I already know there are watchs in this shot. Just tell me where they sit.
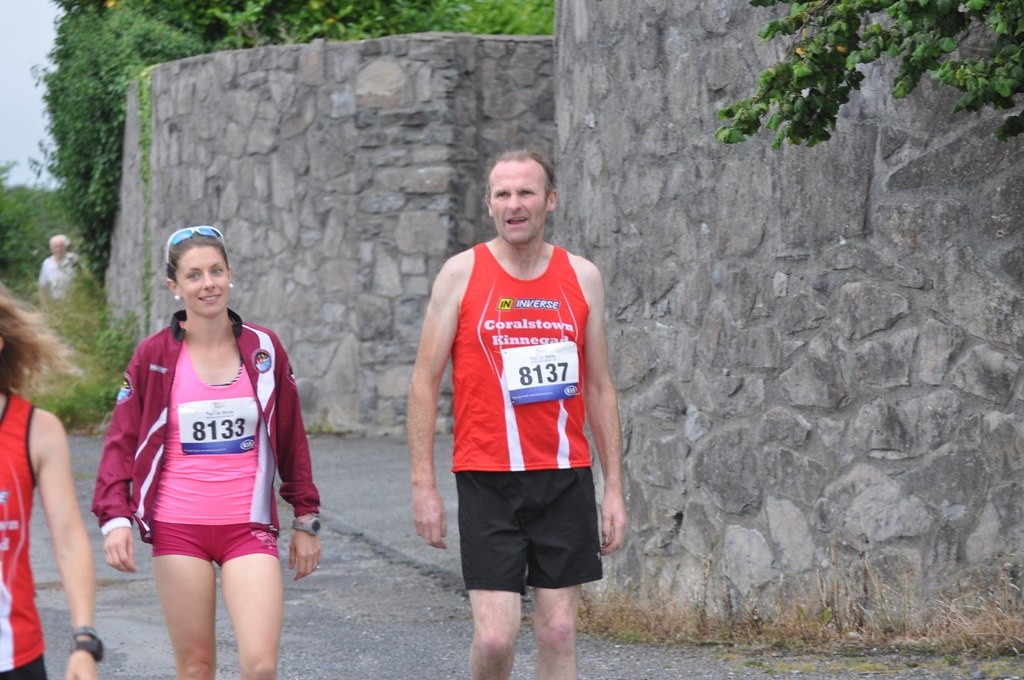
[68,625,103,661]
[290,516,322,535]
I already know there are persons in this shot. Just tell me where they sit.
[404,149,630,680]
[0,283,105,680]
[38,235,99,305]
[91,223,323,679]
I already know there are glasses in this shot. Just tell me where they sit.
[165,226,224,264]
